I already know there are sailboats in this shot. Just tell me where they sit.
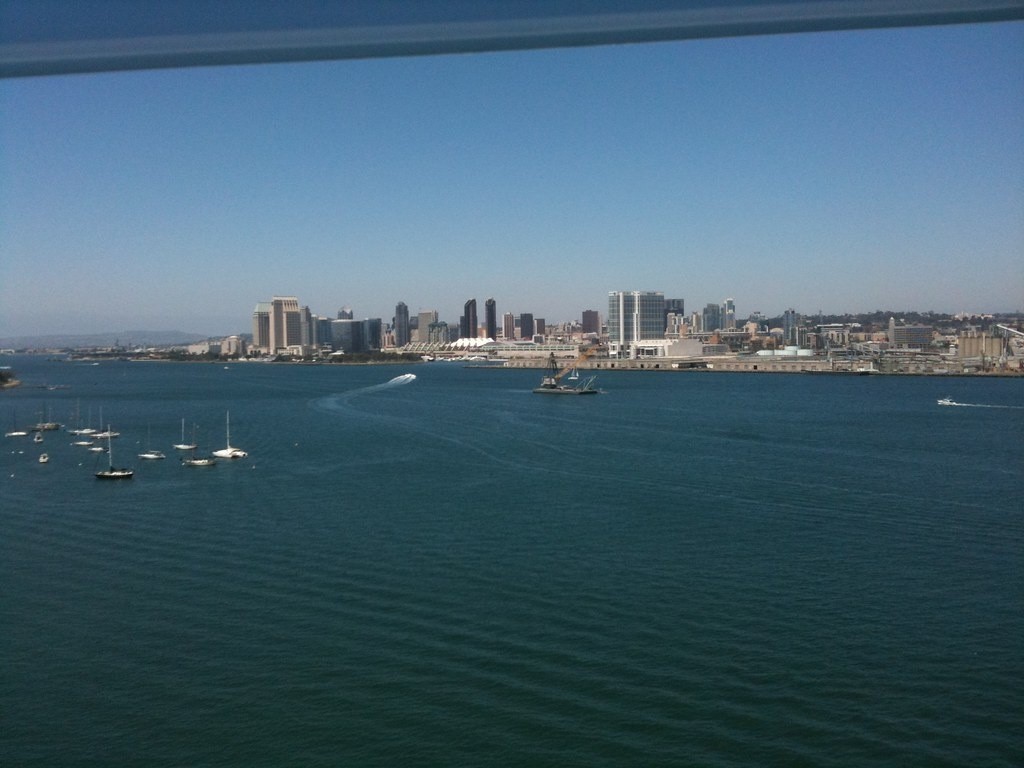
[5,400,251,479]
[567,365,580,380]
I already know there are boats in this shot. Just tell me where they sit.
[533,345,600,393]
